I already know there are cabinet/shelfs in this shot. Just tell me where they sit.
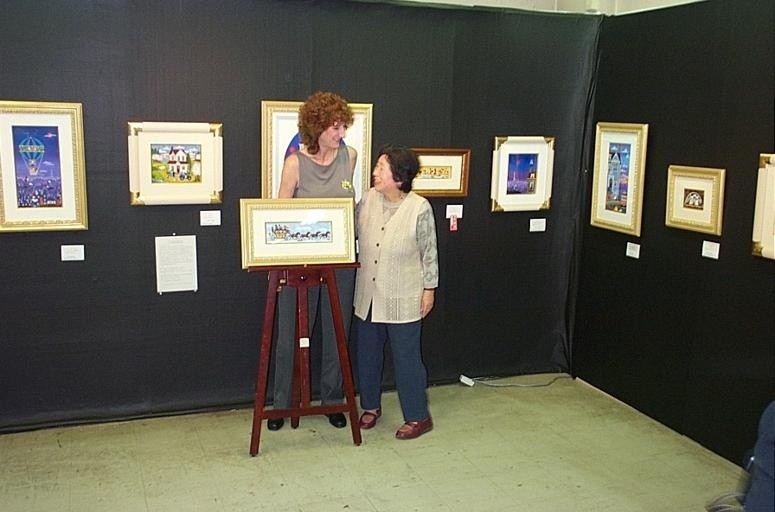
[246,261,362,458]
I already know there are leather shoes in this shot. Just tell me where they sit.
[267,418,284,430]
[360,405,381,429]
[325,413,346,427]
[396,418,433,439]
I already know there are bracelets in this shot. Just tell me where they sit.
[423,288,435,291]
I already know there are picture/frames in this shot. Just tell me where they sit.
[665,164,726,238]
[590,121,650,237]
[261,99,373,203]
[490,135,556,211]
[749,153,775,260]
[409,147,472,198]
[128,121,224,206]
[239,198,356,270]
[0,101,89,232]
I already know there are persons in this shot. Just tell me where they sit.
[352,143,439,440]
[266,89,358,431]
[741,401,775,512]
